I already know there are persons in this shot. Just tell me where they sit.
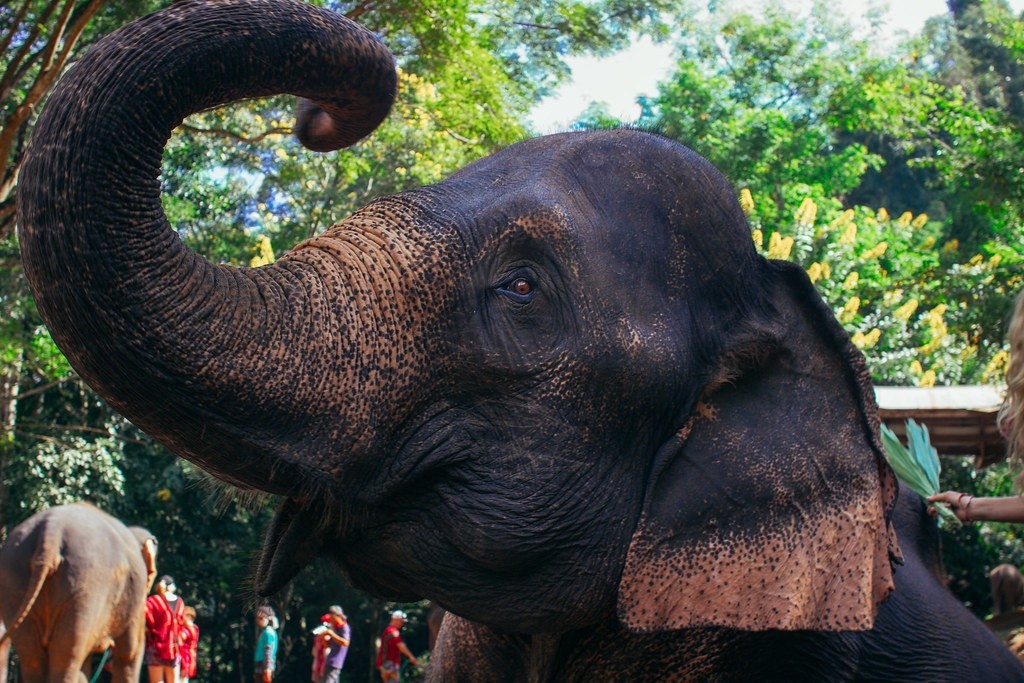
[923,285,1024,529]
[312,605,351,682]
[375,610,424,683]
[253,606,279,683]
[174,606,199,683]
[989,561,1024,666]
[310,614,335,683]
[144,575,185,683]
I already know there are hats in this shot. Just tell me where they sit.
[258,606,275,616]
[391,610,407,619]
[330,605,347,620]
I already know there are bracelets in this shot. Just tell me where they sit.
[958,493,974,523]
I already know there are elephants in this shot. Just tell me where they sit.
[0,500,159,683]
[17,1,1024,682]
